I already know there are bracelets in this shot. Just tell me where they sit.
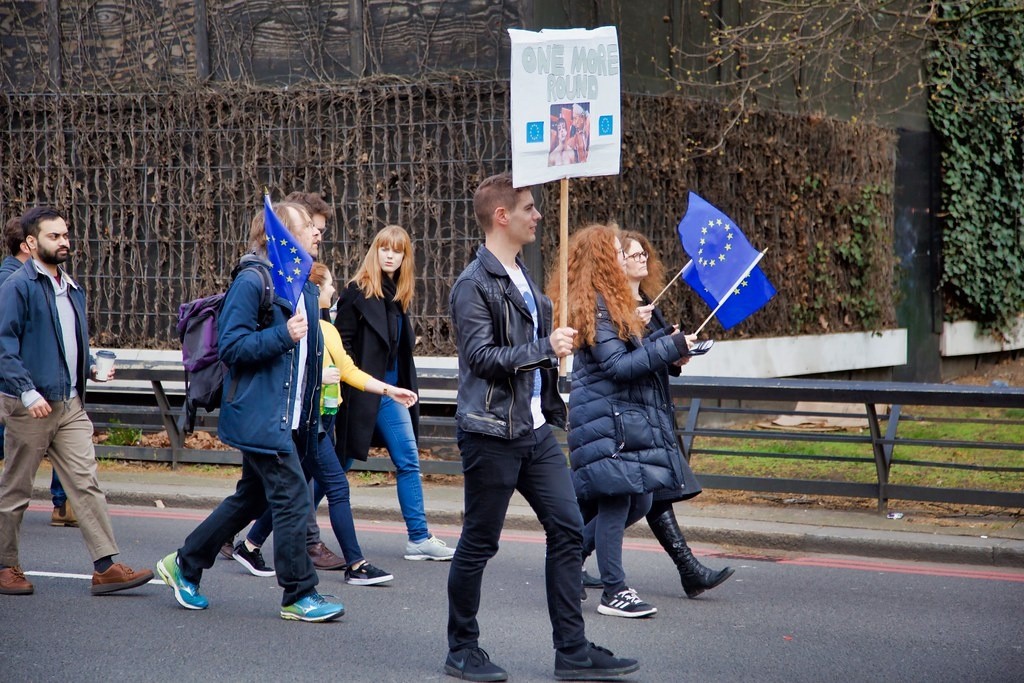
[383,385,388,395]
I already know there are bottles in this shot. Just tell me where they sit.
[323,365,338,415]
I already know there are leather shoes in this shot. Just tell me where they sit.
[307,541,346,568]
[0,565,33,592]
[91,562,154,592]
[220,538,234,559]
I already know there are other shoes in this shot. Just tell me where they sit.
[51,499,78,526]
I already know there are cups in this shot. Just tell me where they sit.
[96,350,116,381]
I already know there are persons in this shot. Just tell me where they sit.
[544,225,698,617]
[220,192,345,571]
[0,207,154,596]
[444,173,639,683]
[156,202,345,624]
[580,234,734,598]
[334,224,457,561]
[230,263,418,586]
[548,104,590,166]
[0,217,80,527]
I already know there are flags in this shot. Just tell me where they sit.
[678,190,759,303]
[263,195,314,317]
[682,262,775,329]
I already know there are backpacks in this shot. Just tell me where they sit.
[176,264,274,408]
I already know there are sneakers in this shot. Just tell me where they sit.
[444,647,508,681]
[281,592,345,622]
[598,586,657,618]
[156,549,208,609]
[580,587,588,603]
[345,561,394,584]
[403,534,457,561]
[554,641,640,679]
[232,542,276,576]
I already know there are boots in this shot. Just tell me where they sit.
[646,498,735,599]
[581,568,602,588]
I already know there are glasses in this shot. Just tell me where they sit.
[626,250,648,261]
[617,248,624,258]
